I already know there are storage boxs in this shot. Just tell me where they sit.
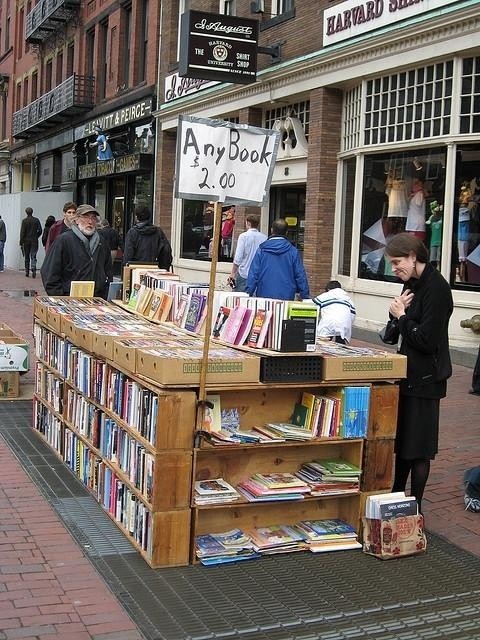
[0,324,30,398]
[31,294,407,382]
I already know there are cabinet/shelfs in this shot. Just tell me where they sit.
[195,384,372,568]
[358,385,403,541]
[32,321,192,572]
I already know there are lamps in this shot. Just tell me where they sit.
[412,153,423,171]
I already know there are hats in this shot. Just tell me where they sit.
[77,204,99,217]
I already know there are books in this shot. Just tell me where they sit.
[195,518,362,566]
[198,387,371,446]
[365,492,418,520]
[36,270,317,359]
[315,335,388,359]
[194,458,363,505]
[33,323,158,561]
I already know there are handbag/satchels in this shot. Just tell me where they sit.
[157,226,172,270]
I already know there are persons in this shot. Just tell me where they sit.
[20,207,42,278]
[230,214,268,294]
[46,201,76,253]
[310,281,357,346]
[384,166,475,264]
[89,127,114,162]
[379,233,454,518]
[245,219,311,302]
[462,464,480,514]
[96,219,119,251]
[122,206,173,271]
[0,220,7,272]
[466,243,479,396]
[40,203,114,299]
[41,216,56,247]
